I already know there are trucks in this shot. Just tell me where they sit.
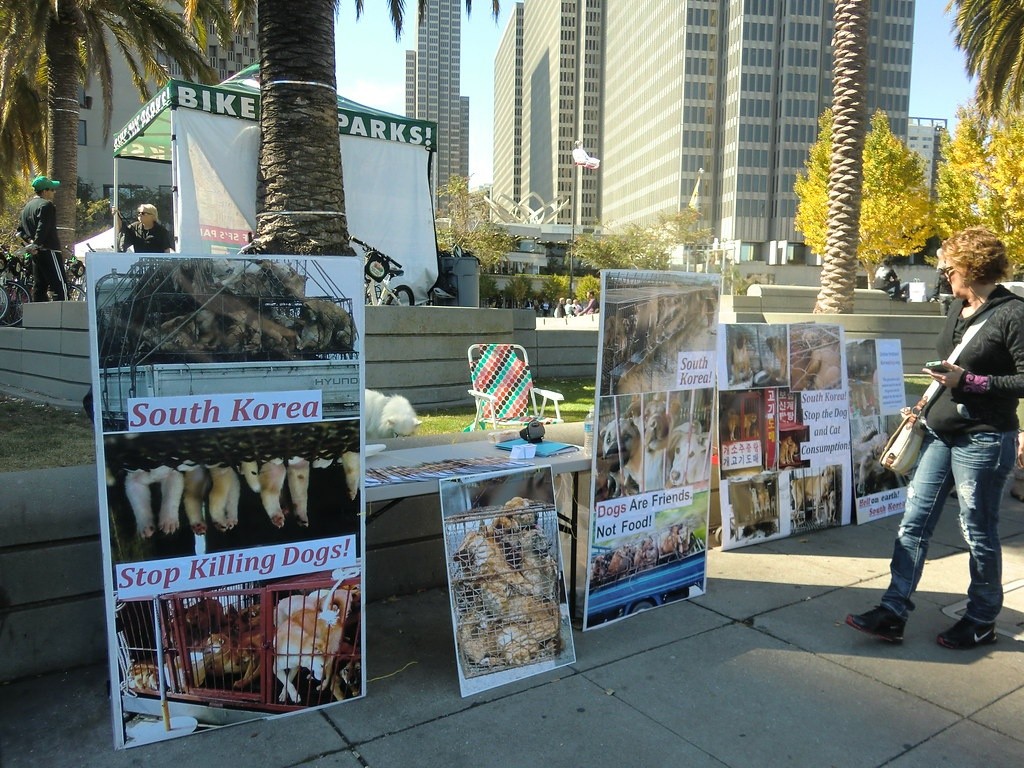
[98,357,360,421]
[587,548,704,617]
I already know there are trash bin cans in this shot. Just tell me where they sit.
[439,254,479,308]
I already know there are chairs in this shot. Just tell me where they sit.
[466,343,566,432]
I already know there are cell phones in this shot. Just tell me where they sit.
[926,360,952,373]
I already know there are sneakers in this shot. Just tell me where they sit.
[937,616,996,648]
[847,605,907,644]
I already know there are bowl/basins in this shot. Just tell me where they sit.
[753,370,773,387]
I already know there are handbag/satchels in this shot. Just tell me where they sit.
[880,409,926,476]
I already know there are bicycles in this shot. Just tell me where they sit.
[0,242,88,327]
[348,236,415,307]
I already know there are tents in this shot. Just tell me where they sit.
[114,65,441,305]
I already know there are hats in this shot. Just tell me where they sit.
[32,176,60,191]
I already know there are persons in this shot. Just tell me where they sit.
[112,204,175,255]
[528,292,599,316]
[874,238,951,302]
[846,227,1024,649]
[17,177,69,301]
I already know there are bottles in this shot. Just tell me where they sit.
[583,404,594,457]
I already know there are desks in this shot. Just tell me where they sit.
[363,440,592,621]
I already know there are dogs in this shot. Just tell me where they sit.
[586,275,718,631]
[729,436,840,544]
[125,588,361,708]
[452,497,561,666]
[728,408,756,441]
[365,388,423,438]
[725,324,843,391]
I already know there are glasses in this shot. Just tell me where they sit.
[137,211,154,216]
[944,265,955,280]
